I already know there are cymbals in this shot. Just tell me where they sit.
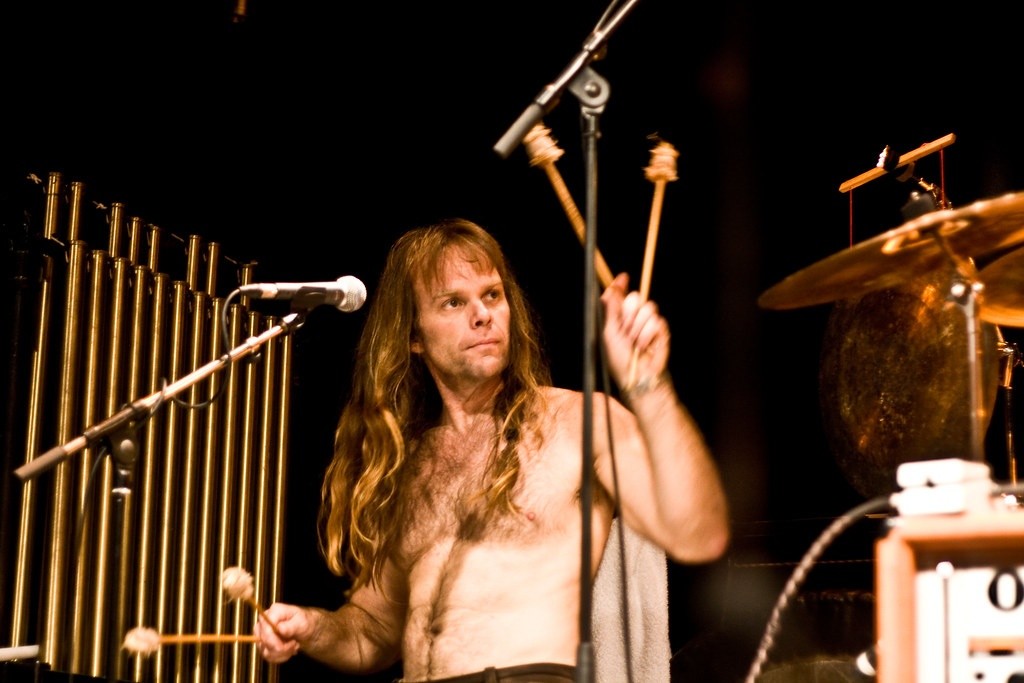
[978,244,1024,328]
[756,188,1024,309]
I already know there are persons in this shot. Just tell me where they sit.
[252,223,730,683]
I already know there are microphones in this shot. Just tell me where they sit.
[239,276,367,313]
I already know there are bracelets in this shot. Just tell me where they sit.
[620,371,670,405]
